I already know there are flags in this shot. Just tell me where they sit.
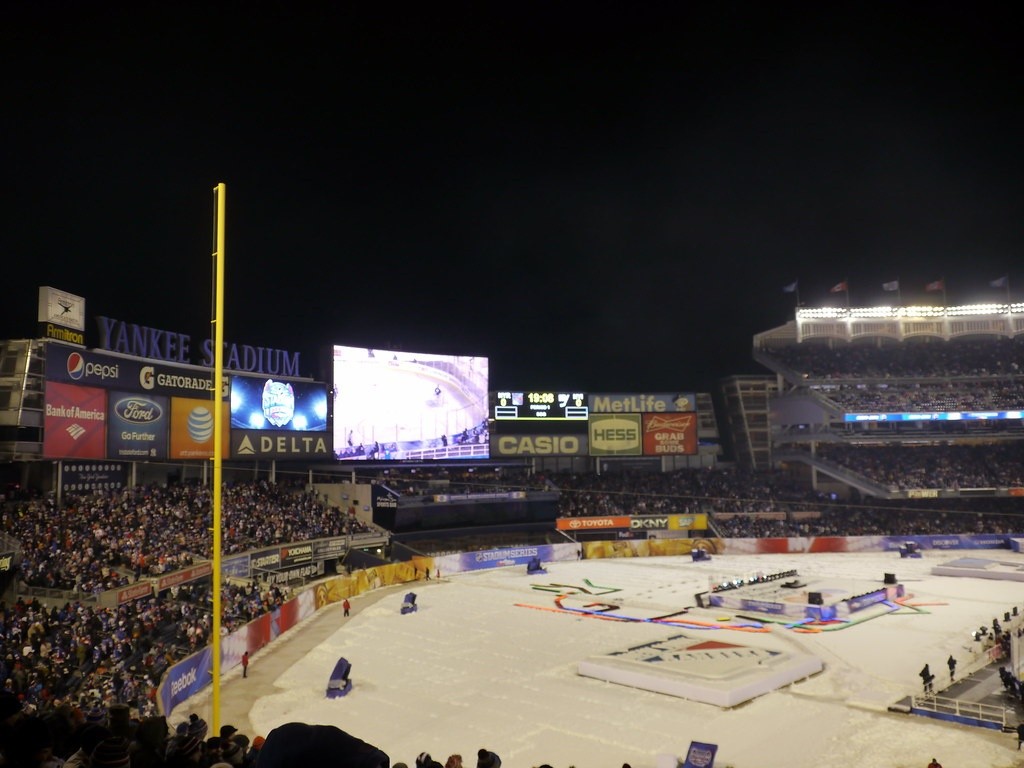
[784,282,797,292]
[926,280,942,291]
[832,281,846,292]
[883,281,898,291]
[991,276,1007,288]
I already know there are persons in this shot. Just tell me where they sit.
[333,355,489,460]
[759,337,1024,488]
[242,651,250,677]
[343,599,350,617]
[0,465,1024,768]
[919,663,933,694]
[947,654,957,681]
[577,549,581,562]
[426,567,431,581]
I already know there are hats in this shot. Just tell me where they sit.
[253,736,266,750]
[221,725,238,739]
[175,734,201,756]
[86,707,105,723]
[89,737,132,768]
[221,740,242,761]
[477,749,501,768]
[188,714,207,736]
[0,690,24,719]
[11,717,54,753]
